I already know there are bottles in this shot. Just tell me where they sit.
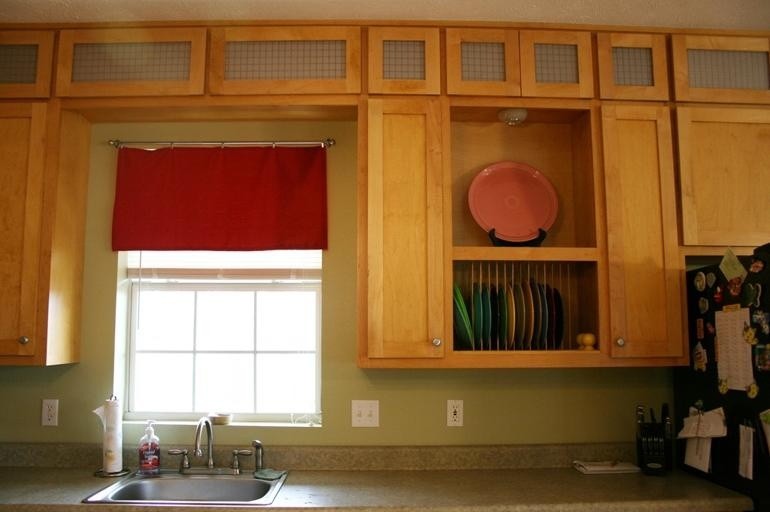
[138,421,162,475]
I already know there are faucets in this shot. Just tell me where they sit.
[194,417,214,469]
[251,440,264,474]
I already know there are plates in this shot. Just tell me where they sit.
[468,161,558,242]
[453,279,574,349]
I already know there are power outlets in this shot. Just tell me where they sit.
[40,398,61,427]
[444,399,465,428]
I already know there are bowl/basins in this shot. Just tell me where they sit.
[208,412,232,425]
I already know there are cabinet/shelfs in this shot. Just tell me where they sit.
[55,20,366,125]
[670,28,770,258]
[0,24,92,368]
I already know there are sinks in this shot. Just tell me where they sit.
[81,468,289,506]
